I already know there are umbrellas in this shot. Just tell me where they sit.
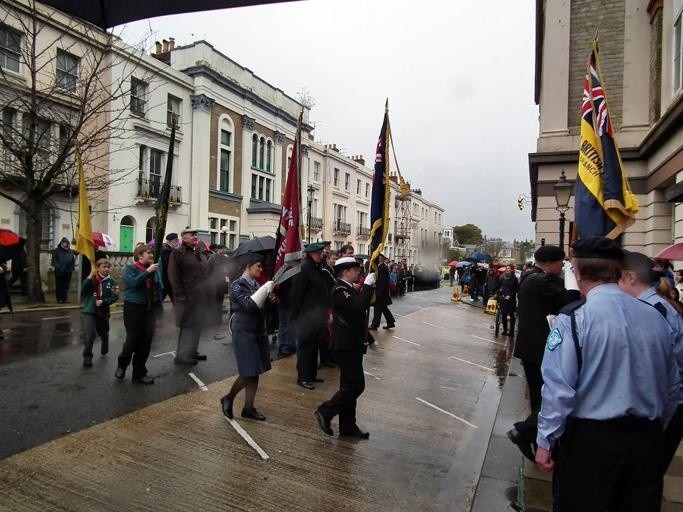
[653,242,683,261]
[0,229,21,246]
[447,251,492,269]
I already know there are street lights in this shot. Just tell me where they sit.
[552,172,572,251]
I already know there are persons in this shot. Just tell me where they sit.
[535,237,678,512]
[506,244,586,463]
[449,263,544,336]
[83,228,231,383]
[384,256,441,298]
[0,237,75,320]
[617,252,683,476]
[651,258,683,318]
[219,240,396,439]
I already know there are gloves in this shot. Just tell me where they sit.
[250,280,275,309]
[504,295,511,302]
[363,273,376,286]
[177,294,186,306]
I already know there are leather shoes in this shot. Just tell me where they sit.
[506,428,535,463]
[191,352,206,360]
[58,300,63,304]
[383,323,395,329]
[132,376,153,384]
[314,410,333,435]
[217,305,227,313]
[297,379,314,389]
[349,429,369,438]
[174,356,197,366]
[241,408,265,421]
[368,326,377,330]
[323,362,336,368]
[279,344,291,356]
[311,376,323,382]
[221,396,234,419]
[63,300,71,303]
[115,368,125,379]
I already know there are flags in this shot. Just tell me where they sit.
[271,114,307,287]
[367,112,390,273]
[153,126,176,263]
[574,35,639,240]
[75,158,97,273]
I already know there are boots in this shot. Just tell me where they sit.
[501,316,507,336]
[507,318,515,336]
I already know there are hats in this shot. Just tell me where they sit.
[304,243,324,252]
[534,246,565,262]
[379,254,385,258]
[332,257,360,271]
[166,233,177,240]
[570,237,624,262]
[209,244,218,250]
[218,243,224,249]
[181,229,198,237]
[622,250,656,270]
[239,253,265,264]
[322,241,331,246]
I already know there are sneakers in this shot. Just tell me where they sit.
[101,342,108,354]
[84,357,92,366]
[369,341,378,350]
